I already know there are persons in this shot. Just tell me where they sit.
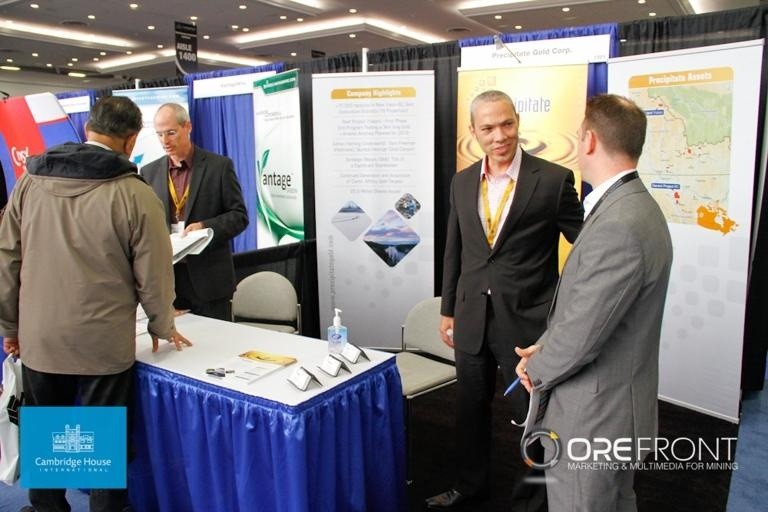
[423,88,585,510]
[139,101,251,322]
[0,94,193,511]
[514,94,675,510]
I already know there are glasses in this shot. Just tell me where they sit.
[155,128,178,138]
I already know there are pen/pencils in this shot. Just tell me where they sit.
[504,369,528,396]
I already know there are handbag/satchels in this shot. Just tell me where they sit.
[0,351,28,485]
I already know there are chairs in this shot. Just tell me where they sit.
[230,271,302,337]
[394,296,461,461]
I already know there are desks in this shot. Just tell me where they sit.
[75,305,409,512]
